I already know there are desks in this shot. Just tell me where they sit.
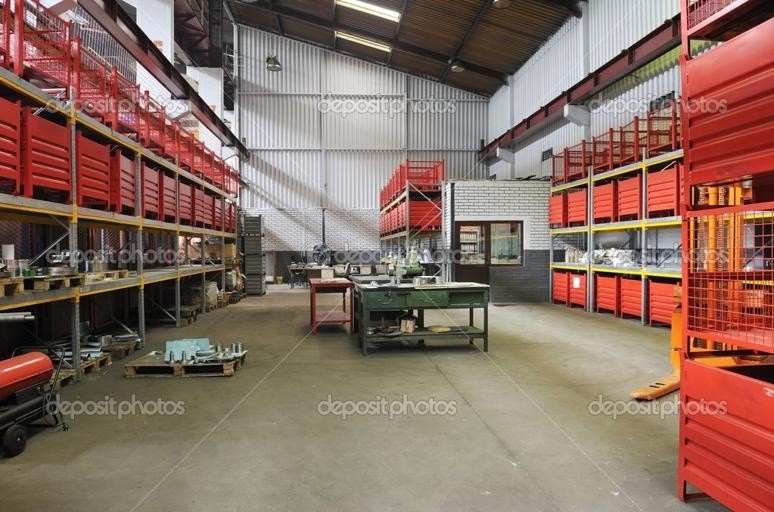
[355,282,490,356]
[549,147,774,326]
[311,278,355,335]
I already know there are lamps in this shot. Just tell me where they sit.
[266,10,281,71]
[451,53,464,73]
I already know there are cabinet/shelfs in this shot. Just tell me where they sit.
[0,66,238,385]
[379,180,446,282]
